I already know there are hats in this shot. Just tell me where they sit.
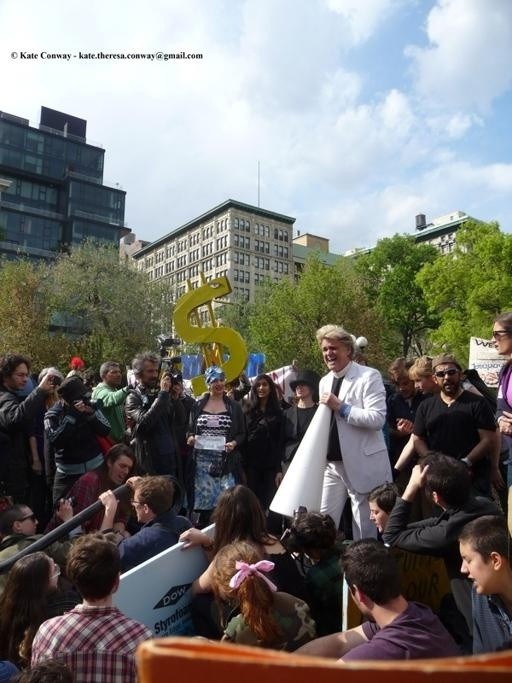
[289,369,320,393]
[204,366,225,385]
[431,352,462,371]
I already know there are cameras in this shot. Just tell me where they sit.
[82,397,103,410]
[50,376,58,385]
[165,373,182,387]
[286,506,307,552]
[56,496,78,512]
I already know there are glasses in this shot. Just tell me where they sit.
[435,369,458,378]
[50,562,62,580]
[492,330,506,337]
[21,512,36,524]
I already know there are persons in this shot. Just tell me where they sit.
[3,312,512,683]
